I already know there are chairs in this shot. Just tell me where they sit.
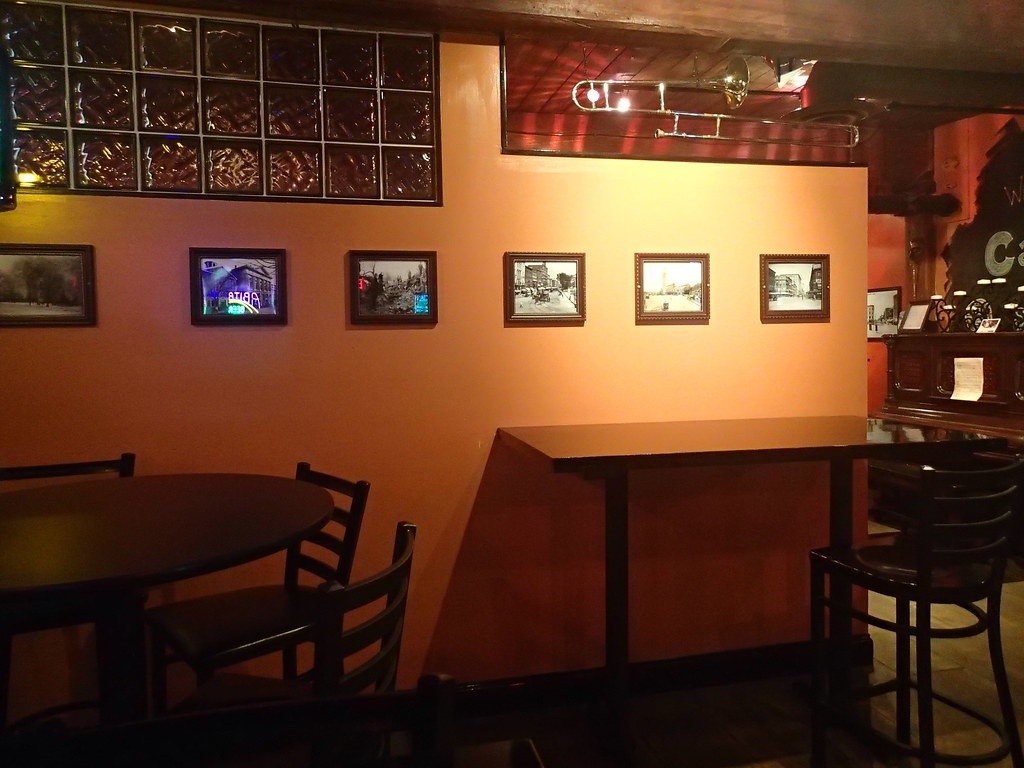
[807,454,1024,768]
[145,461,370,717]
[124,521,417,768]
[1,451,134,710]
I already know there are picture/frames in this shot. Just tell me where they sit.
[635,251,711,326]
[760,253,830,323]
[189,246,288,327]
[348,250,438,325]
[867,286,903,341]
[504,251,586,325]
[0,242,97,327]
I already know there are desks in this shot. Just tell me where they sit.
[0,472,335,768]
[499,418,1009,767]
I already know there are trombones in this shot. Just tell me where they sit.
[572,57,859,148]
